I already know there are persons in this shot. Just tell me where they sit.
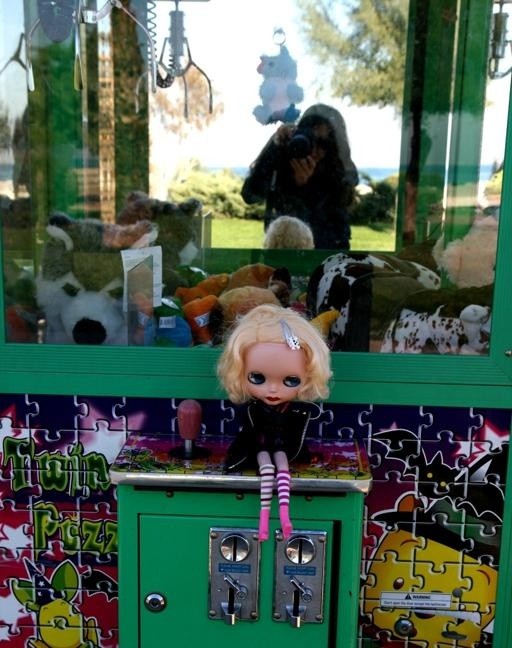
[217,304,335,543]
[241,99,357,249]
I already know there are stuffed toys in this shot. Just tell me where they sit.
[5,191,499,355]
[252,44,303,125]
[6,192,499,356]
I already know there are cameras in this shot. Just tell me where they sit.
[282,126,314,163]
